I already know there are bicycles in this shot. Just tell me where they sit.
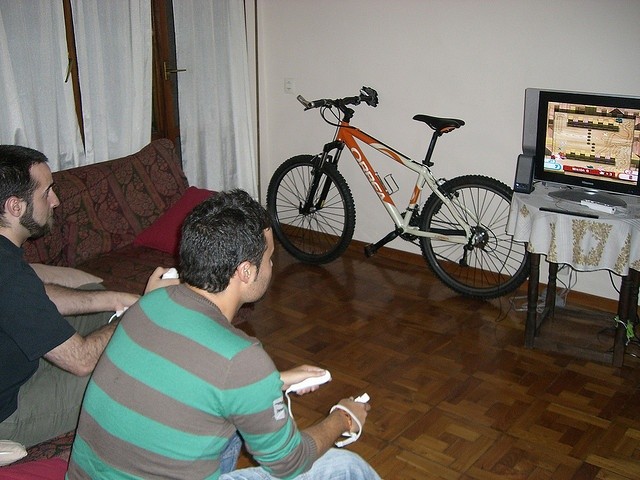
[267,86,532,298]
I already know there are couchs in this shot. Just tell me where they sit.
[0,428,76,480]
[23,139,225,296]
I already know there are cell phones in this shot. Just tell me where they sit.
[513,154,535,194]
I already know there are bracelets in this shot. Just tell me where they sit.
[337,406,354,435]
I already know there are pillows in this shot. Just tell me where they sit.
[0,458,68,480]
[135,187,219,252]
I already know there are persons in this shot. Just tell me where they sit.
[62,190,385,479]
[0,142,182,458]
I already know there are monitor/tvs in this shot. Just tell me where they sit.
[533,91,639,207]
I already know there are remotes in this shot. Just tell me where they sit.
[580,199,617,214]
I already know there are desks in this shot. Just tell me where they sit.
[507,182,640,371]
